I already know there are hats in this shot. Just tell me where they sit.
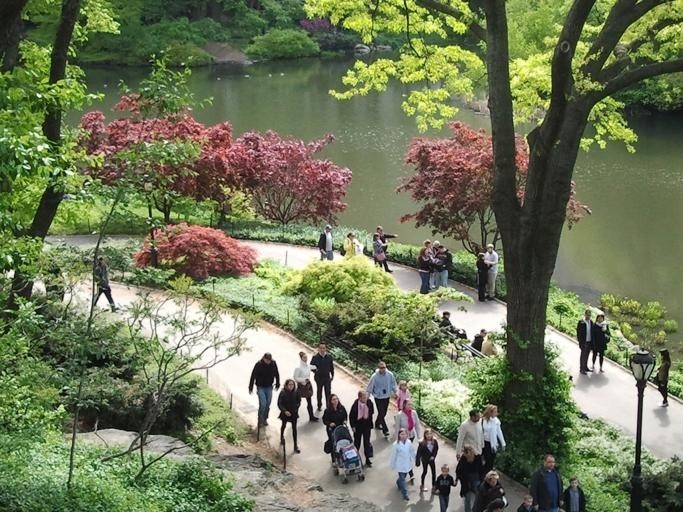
[484,242,495,250]
[323,225,333,230]
[432,239,440,248]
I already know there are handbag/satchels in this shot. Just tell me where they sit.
[324,438,332,453]
[599,332,611,343]
[297,381,313,398]
[375,251,388,261]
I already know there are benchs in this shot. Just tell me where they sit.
[439,329,466,361]
[457,342,486,364]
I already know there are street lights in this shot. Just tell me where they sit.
[140,173,159,271]
[626,346,655,512]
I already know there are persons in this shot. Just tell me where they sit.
[91,257,119,312]
[373,233,393,272]
[476,253,492,302]
[656,349,671,407]
[376,226,398,267]
[417,240,452,294]
[343,232,361,261]
[318,225,333,260]
[484,243,498,301]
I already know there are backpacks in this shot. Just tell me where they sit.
[339,239,349,255]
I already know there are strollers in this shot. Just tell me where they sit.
[323,422,365,485]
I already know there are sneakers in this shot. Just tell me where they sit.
[111,307,120,312]
[395,476,439,501]
[330,459,374,469]
[475,294,495,304]
[384,268,394,274]
[280,438,302,453]
[419,284,437,294]
[308,407,322,422]
[255,418,270,428]
[577,365,608,377]
[374,426,390,437]
[658,402,669,408]
